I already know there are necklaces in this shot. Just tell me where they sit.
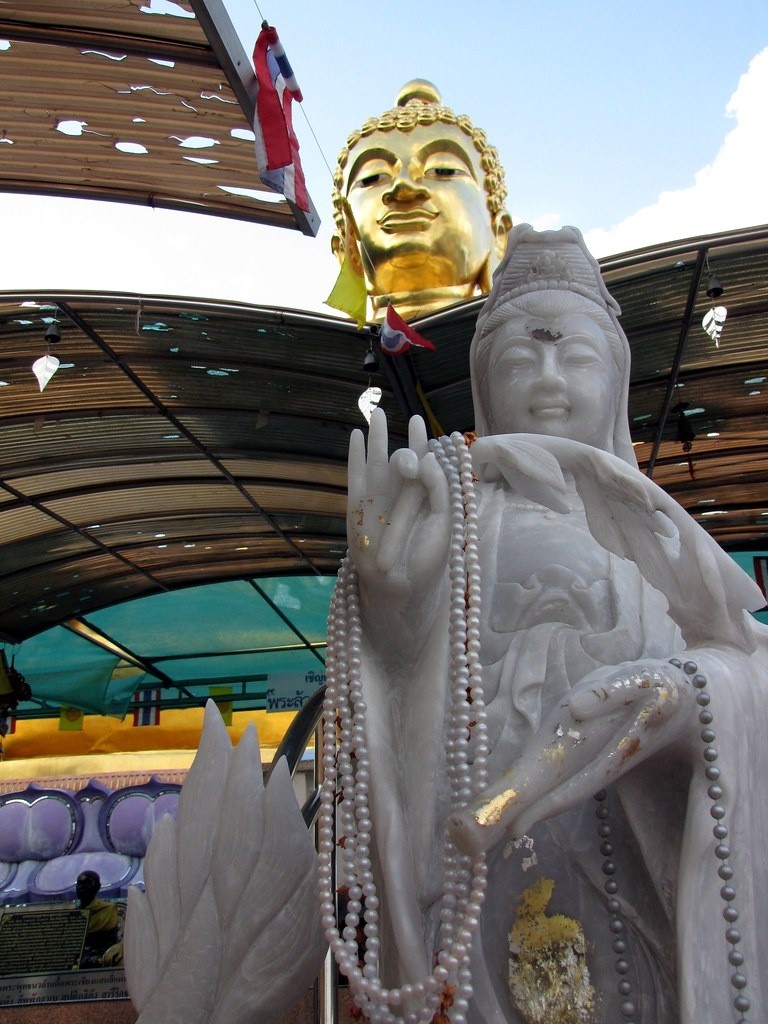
[317,429,490,1024]
[595,657,751,1024]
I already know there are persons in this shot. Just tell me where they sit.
[331,77,512,322]
[342,224,767,1024]
[73,870,123,968]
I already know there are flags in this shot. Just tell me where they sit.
[253,22,312,215]
[380,301,436,355]
[324,209,367,329]
